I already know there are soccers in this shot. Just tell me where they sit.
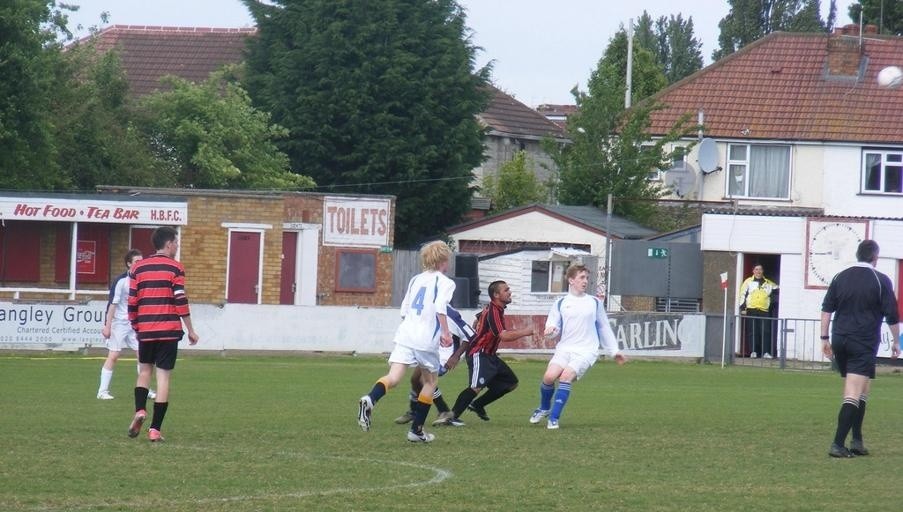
[878,65,902,89]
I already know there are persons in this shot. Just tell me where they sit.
[740,263,779,359]
[97,248,156,399]
[358,240,456,442]
[821,240,901,458]
[394,302,478,424]
[529,265,626,430]
[450,281,535,426]
[127,227,199,442]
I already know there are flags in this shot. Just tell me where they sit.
[720,272,728,289]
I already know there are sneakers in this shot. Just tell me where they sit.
[829,442,853,457]
[148,390,157,401]
[128,410,148,438]
[547,415,560,431]
[395,401,490,443]
[97,392,115,400]
[529,407,551,424]
[148,428,161,442]
[763,352,772,358]
[750,352,757,359]
[850,440,869,456]
[358,395,373,432]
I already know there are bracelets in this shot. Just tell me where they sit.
[820,335,829,340]
